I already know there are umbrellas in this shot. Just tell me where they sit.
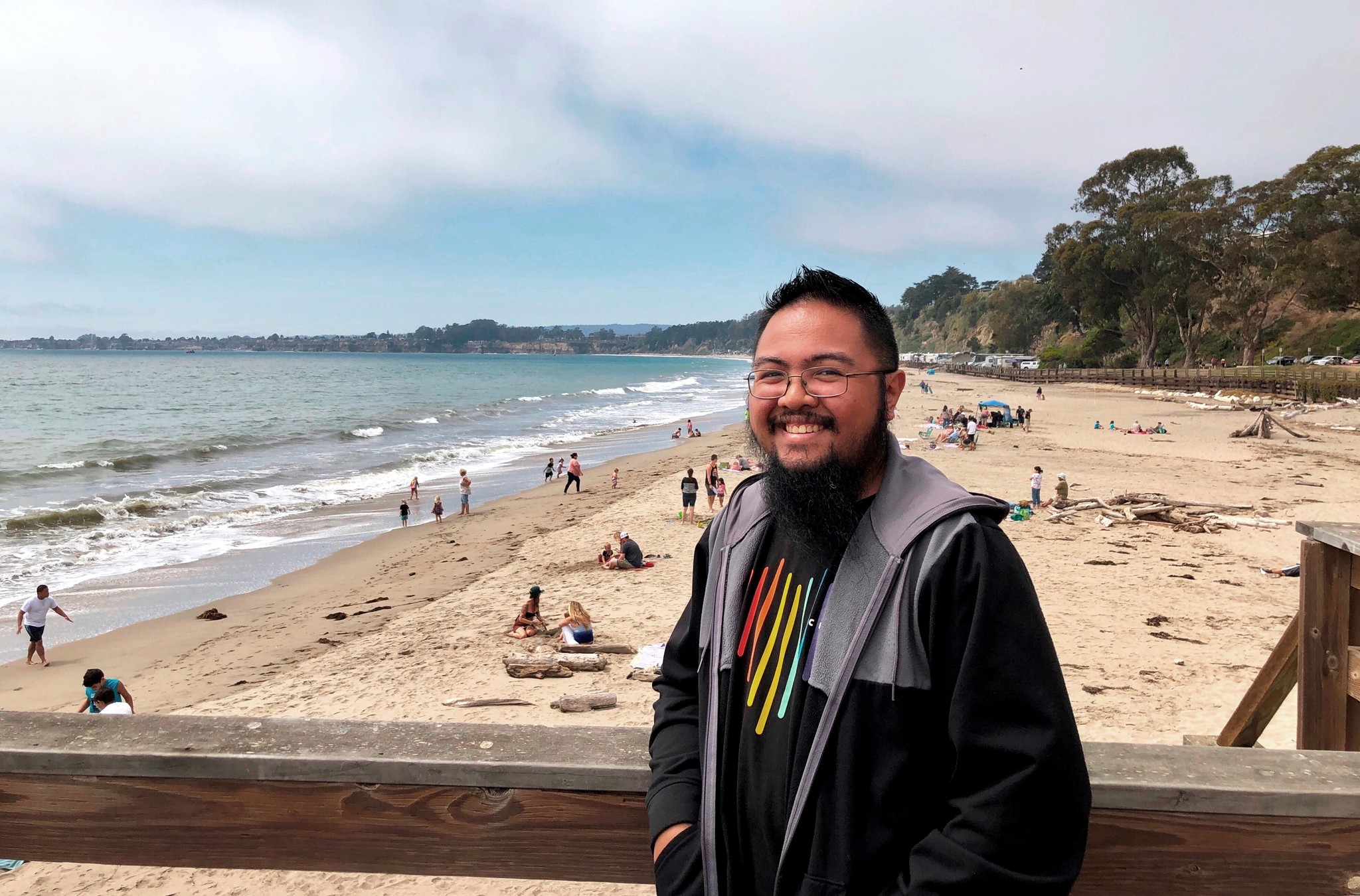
[1007,358,1017,369]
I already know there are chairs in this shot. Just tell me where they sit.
[947,438,959,443]
[918,427,933,439]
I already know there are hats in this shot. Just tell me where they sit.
[948,423,953,427]
[620,532,629,539]
[560,457,564,459]
[961,412,965,415]
[529,586,543,595]
[1057,472,1066,480]
[735,455,739,459]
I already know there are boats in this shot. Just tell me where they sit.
[186,350,195,353]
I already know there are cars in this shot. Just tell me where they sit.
[1312,356,1350,366]
[1345,355,1360,365]
[1266,356,1297,366]
[1296,356,1324,365]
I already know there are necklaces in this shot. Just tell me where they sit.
[38,596,45,606]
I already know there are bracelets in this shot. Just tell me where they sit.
[542,620,545,621]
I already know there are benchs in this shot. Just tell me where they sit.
[1203,363,1212,369]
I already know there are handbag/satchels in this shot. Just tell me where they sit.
[1010,514,1023,520]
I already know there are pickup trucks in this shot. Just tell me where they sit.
[1020,361,1040,370]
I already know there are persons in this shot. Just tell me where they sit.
[564,453,583,495]
[431,496,444,524]
[556,601,594,645]
[892,380,1164,450]
[644,262,1094,896]
[457,469,471,516]
[611,468,620,489]
[409,476,420,500]
[94,686,132,714]
[556,457,566,479]
[671,407,756,525]
[598,531,643,570]
[1059,362,1067,370]
[1145,355,1225,370]
[1029,466,1044,510]
[77,669,135,714]
[399,499,410,527]
[16,585,73,666]
[1041,472,1068,507]
[543,458,556,482]
[503,586,546,640]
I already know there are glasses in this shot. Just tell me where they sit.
[743,366,894,399]
